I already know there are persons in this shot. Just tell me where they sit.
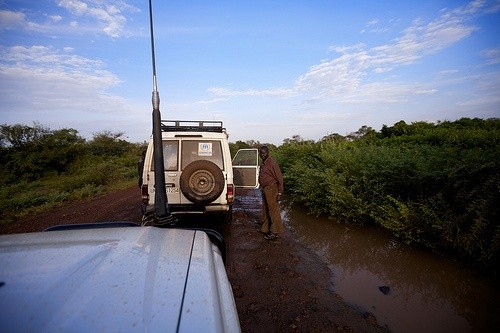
[257,146,283,239]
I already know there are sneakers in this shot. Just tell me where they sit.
[256,228,262,232]
[263,233,279,239]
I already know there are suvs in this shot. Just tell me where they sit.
[139,120,258,217]
